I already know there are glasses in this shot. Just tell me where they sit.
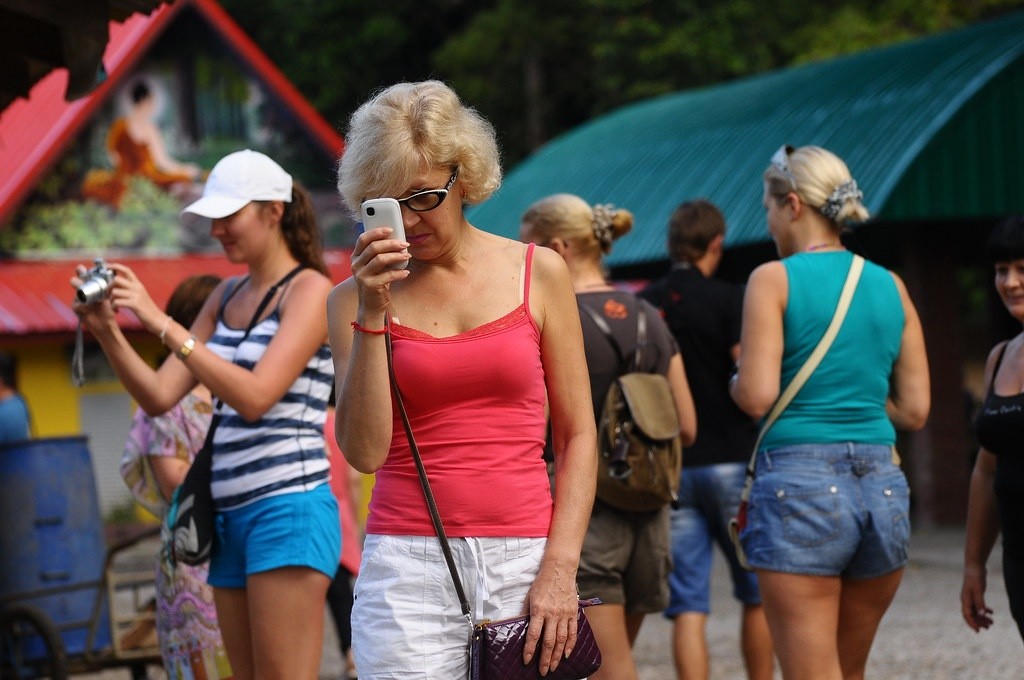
[362,162,461,211]
[770,144,796,198]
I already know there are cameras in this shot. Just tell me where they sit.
[77,258,117,306]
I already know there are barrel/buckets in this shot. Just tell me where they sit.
[0,433,113,661]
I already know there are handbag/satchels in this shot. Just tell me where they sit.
[170,444,215,565]
[727,470,757,573]
[469,599,605,680]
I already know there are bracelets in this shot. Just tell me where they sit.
[177,337,200,361]
[159,316,174,345]
[352,321,388,334]
[728,374,738,384]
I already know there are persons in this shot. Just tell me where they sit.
[730,143,932,680]
[119,274,235,680]
[71,149,342,680]
[634,198,762,680]
[960,206,1024,641]
[326,79,599,680]
[521,194,697,680]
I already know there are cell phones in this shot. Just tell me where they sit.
[361,198,409,273]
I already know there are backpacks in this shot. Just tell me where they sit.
[574,294,680,515]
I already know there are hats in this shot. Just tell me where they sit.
[179,145,292,222]
